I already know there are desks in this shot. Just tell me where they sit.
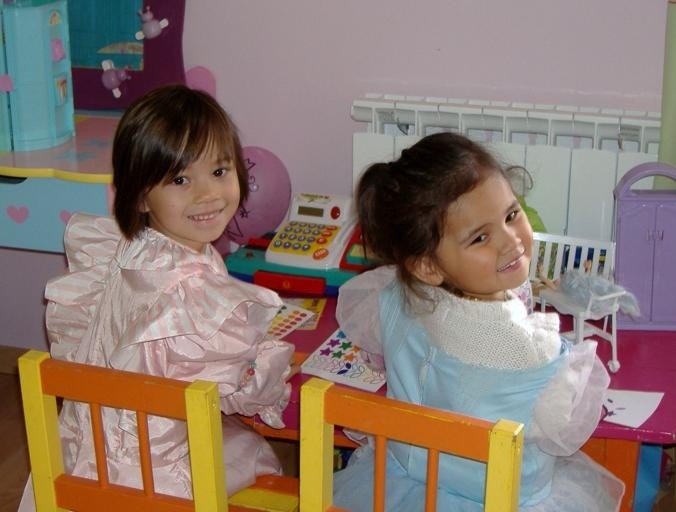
[199,264,673,510]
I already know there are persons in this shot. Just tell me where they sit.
[16,86,295,511]
[334,132,626,511]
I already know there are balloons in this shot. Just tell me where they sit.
[217,145,292,246]
